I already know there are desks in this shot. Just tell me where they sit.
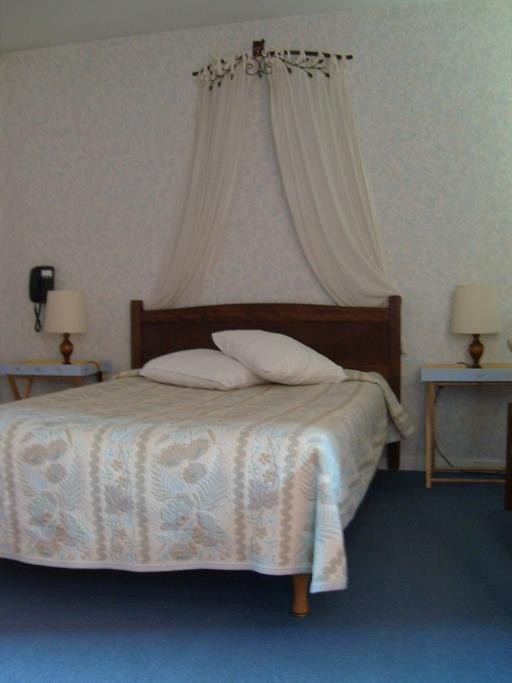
[1,359,111,400]
[418,362,512,489]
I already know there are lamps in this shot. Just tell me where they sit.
[44,289,91,364]
[449,282,502,368]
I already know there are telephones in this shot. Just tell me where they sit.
[29,266,54,303]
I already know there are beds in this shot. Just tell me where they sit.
[1,295,403,618]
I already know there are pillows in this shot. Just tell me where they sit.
[138,327,347,393]
